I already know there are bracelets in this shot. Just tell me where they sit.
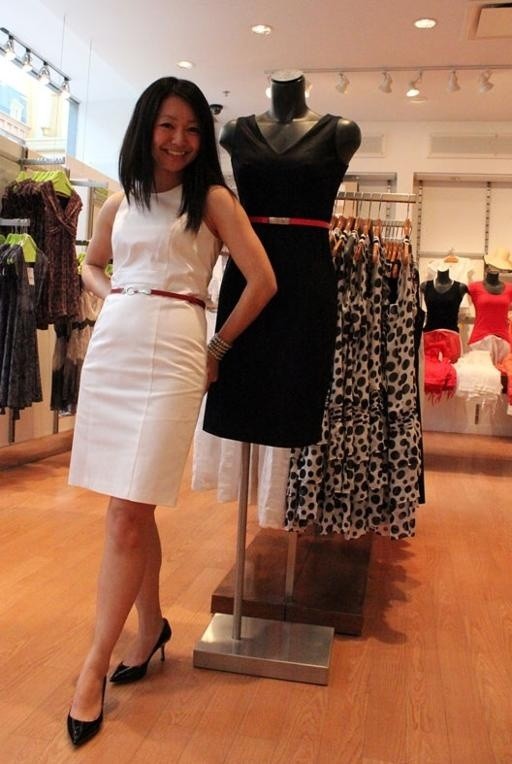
[208,333,231,361]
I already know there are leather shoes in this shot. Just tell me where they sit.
[66,675,107,746]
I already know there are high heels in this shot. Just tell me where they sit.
[108,617,173,684]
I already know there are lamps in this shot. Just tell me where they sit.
[262,64,501,102]
[0,25,72,96]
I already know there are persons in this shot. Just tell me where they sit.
[201,70,361,448]
[419,267,467,363]
[67,77,278,748]
[466,270,512,366]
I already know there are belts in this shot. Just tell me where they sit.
[110,287,207,311]
[247,216,331,230]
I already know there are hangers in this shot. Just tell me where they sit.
[319,188,420,280]
[71,239,116,278]
[0,217,52,266]
[430,246,472,266]
[8,157,82,204]
